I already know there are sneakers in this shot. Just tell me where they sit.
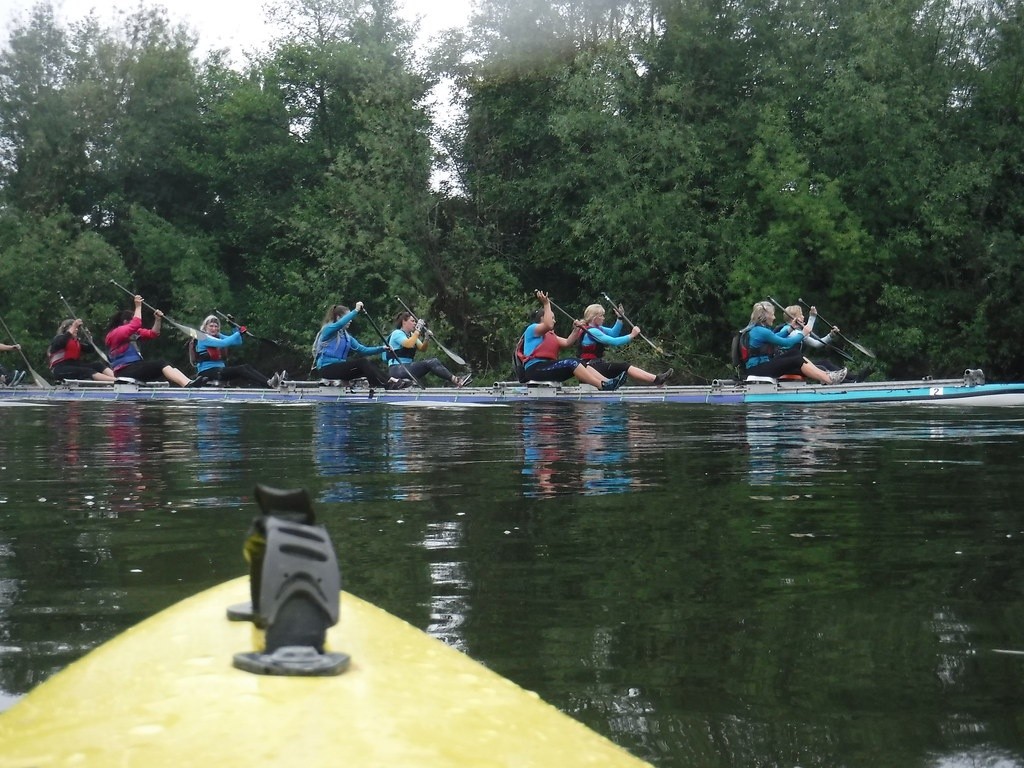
[4,370,26,386]
[600,371,626,391]
[828,367,847,385]
[185,376,209,388]
[268,370,288,388]
[386,378,412,389]
[654,368,673,387]
[458,372,474,387]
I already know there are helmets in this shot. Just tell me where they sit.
[61,319,74,326]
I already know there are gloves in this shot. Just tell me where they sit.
[424,330,433,340]
[226,314,236,328]
[415,319,426,331]
[240,326,247,333]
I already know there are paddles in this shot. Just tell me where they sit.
[109,278,208,342]
[0,316,52,389]
[57,290,112,366]
[211,306,280,348]
[393,294,467,365]
[360,306,427,392]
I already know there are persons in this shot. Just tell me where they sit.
[48,319,116,381]
[0,343,27,388]
[742,301,847,386]
[105,294,209,388]
[524,291,626,391]
[316,301,413,390]
[387,311,473,388]
[197,314,287,389]
[580,303,674,387]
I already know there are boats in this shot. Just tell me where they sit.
[2,374,1024,406]
